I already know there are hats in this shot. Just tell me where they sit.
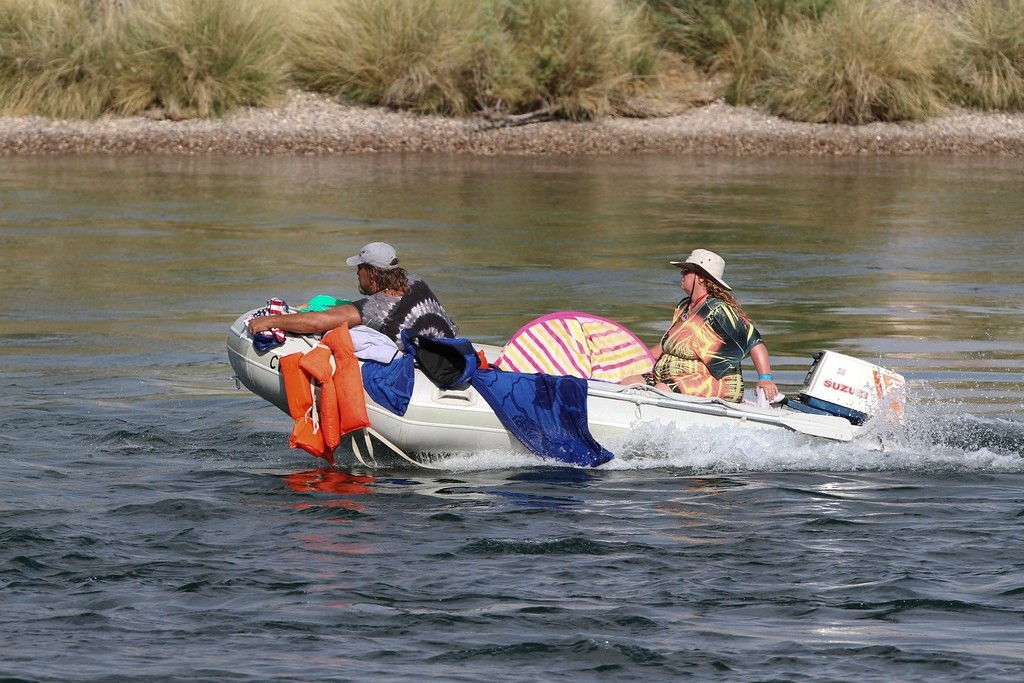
[345,241,400,270]
[669,249,733,291]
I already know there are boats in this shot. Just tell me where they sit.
[227,304,895,471]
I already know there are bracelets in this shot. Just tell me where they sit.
[758,374,774,380]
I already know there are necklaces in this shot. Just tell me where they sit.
[681,293,708,322]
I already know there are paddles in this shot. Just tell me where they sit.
[587,386,855,442]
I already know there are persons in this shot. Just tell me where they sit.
[619,249,779,402]
[249,242,459,354]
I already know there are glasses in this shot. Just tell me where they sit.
[679,266,693,276]
